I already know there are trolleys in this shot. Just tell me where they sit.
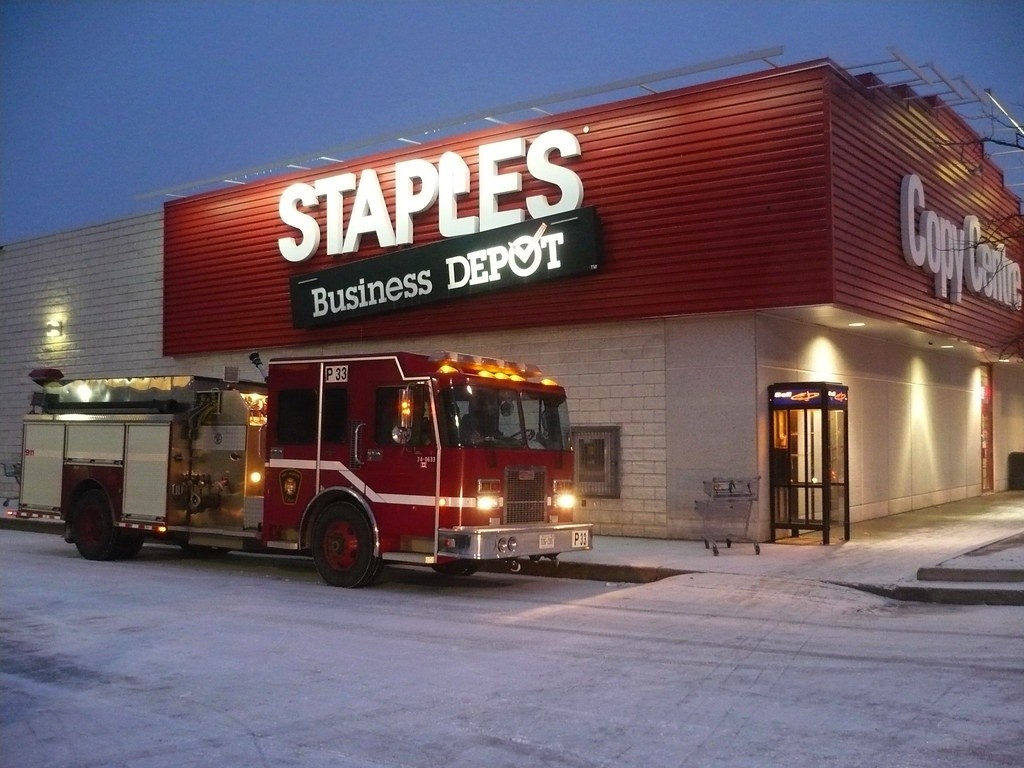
[693,473,762,556]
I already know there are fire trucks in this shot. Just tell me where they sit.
[16,349,597,590]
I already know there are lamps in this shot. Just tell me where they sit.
[46,321,62,337]
